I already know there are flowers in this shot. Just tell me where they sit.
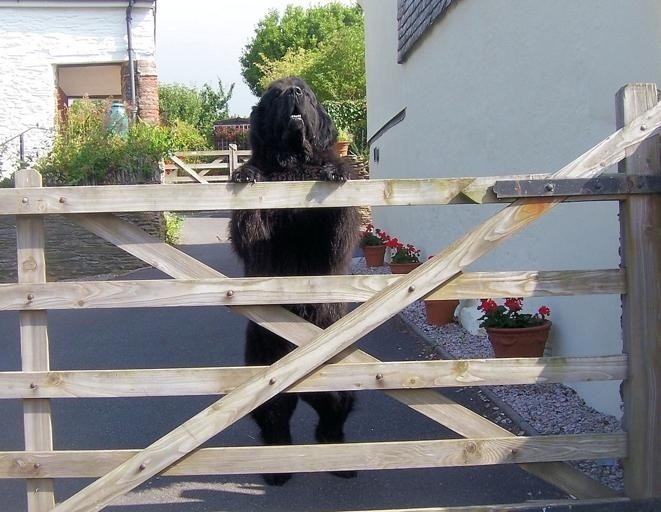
[478,297,552,329]
[358,224,421,263]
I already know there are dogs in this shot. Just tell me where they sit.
[225,74,364,487]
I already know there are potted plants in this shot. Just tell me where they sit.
[331,127,354,157]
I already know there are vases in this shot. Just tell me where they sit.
[486,320,552,358]
[389,264,421,274]
[424,300,461,326]
[362,245,386,267]
